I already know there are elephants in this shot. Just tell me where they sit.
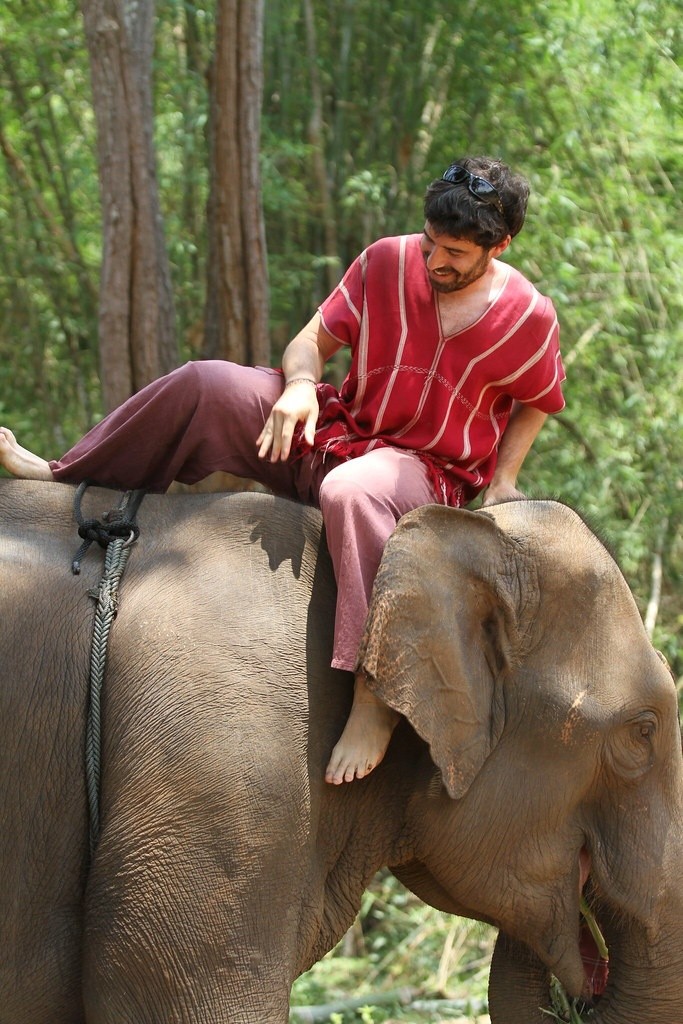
[0,434,683,1024]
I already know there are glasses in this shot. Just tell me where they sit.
[442,164,506,220]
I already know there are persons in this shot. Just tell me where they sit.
[1,156,566,785]
